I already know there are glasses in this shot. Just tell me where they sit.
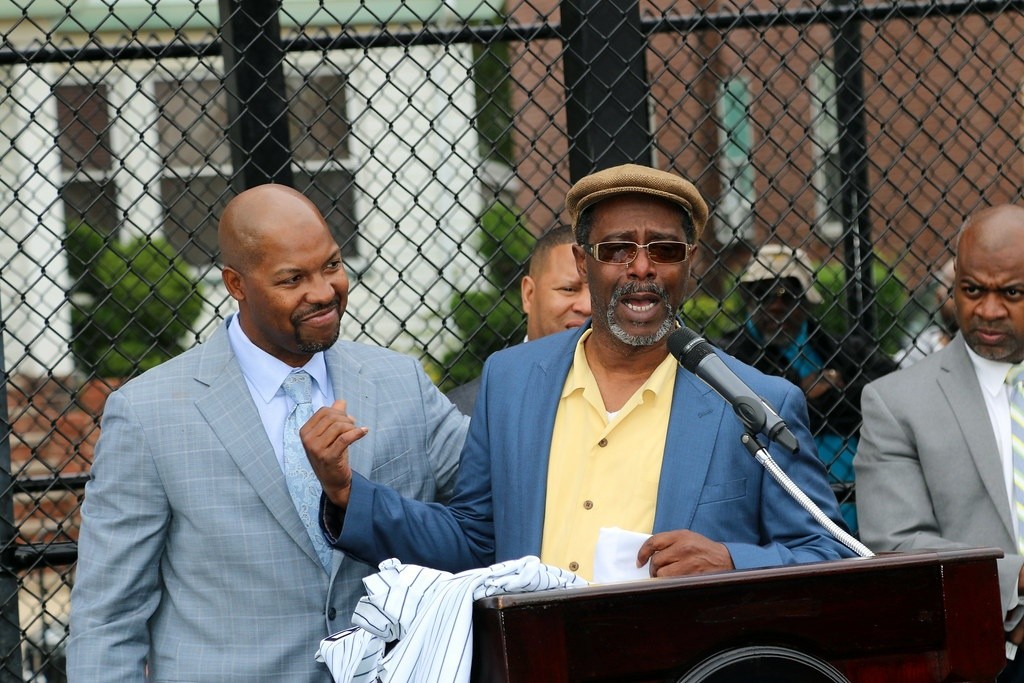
[747,286,803,302]
[579,239,693,265]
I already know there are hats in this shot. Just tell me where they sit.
[565,164,709,241]
[737,245,826,306]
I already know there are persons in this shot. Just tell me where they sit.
[710,243,861,557]
[853,203,1024,683]
[445,224,591,417]
[66,183,472,683]
[893,256,960,369]
[298,163,861,577]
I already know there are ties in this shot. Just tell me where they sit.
[281,370,330,576]
[1004,365,1024,555]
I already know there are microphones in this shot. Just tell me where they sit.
[666,327,800,453]
